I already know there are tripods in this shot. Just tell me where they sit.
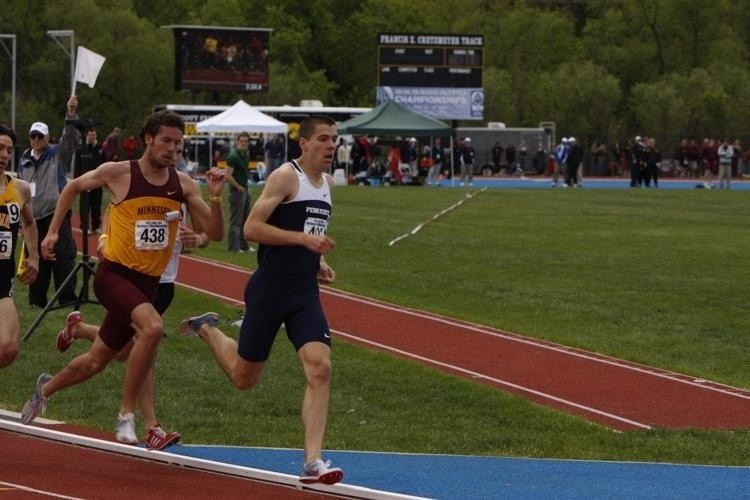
[16,141,98,343]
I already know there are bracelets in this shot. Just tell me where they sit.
[97,234,108,241]
[197,234,204,247]
[319,260,328,266]
[208,195,224,204]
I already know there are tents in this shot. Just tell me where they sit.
[193,100,291,180]
[335,100,456,190]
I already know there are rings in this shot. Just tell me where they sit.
[192,234,194,238]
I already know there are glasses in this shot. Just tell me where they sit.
[30,134,44,139]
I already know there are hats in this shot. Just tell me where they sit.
[28,121,50,137]
[464,136,472,143]
[561,136,576,143]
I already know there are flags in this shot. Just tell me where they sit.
[74,45,106,89]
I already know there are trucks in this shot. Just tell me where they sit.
[454,125,553,177]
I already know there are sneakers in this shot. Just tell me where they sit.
[56,311,83,352]
[179,310,219,337]
[115,411,182,453]
[459,182,474,188]
[298,460,344,486]
[551,180,585,189]
[19,371,54,426]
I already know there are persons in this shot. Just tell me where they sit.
[0,126,40,369]
[17,94,80,309]
[590,134,749,189]
[179,115,344,485]
[175,128,305,253]
[491,140,548,175]
[20,110,226,444]
[334,128,476,187]
[550,136,584,189]
[71,123,140,235]
[57,136,209,451]
